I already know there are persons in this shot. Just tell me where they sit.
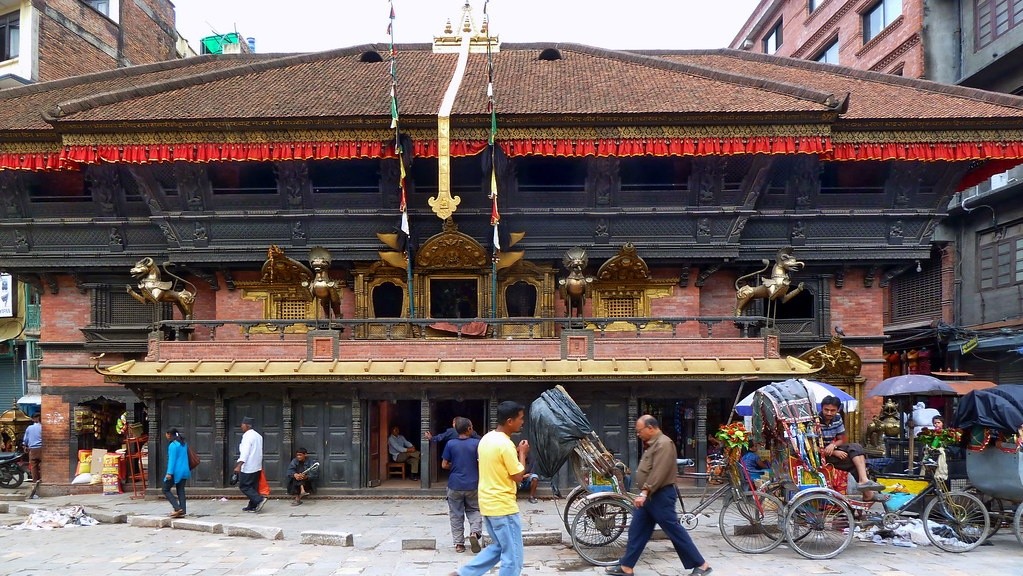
[233,416,268,513]
[738,439,782,483]
[605,448,632,492]
[162,427,192,518]
[286,447,321,506]
[448,401,529,576]
[931,415,945,431]
[604,414,713,576]
[811,395,891,502]
[387,425,419,481]
[425,417,482,554]
[514,446,539,504]
[22,412,43,483]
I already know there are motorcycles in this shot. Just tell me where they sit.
[0,451,30,489]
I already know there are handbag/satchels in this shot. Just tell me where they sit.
[259,470,270,496]
[183,438,200,470]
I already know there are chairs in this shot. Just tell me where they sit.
[734,462,772,500]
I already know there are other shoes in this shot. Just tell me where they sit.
[255,498,267,512]
[171,511,186,517]
[411,476,417,480]
[449,572,459,576]
[528,497,538,503]
[470,536,481,553]
[456,545,466,553]
[242,508,256,513]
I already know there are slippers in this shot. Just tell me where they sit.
[688,566,712,576]
[862,492,890,501]
[605,564,634,576]
[291,501,303,505]
[856,480,886,491]
[300,492,310,499]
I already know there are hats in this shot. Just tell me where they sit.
[242,416,256,424]
[917,402,925,409]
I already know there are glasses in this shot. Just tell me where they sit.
[634,426,648,433]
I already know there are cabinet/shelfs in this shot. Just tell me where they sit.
[124,435,148,500]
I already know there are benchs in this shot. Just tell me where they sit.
[572,452,619,492]
[782,444,837,489]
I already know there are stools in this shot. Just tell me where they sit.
[386,462,406,480]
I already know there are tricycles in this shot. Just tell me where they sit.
[753,377,990,560]
[948,384,1023,545]
[528,385,785,566]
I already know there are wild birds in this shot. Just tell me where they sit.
[835,326,845,336]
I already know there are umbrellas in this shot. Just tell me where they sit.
[864,374,957,419]
[733,378,859,419]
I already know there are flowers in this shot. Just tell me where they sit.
[917,426,962,447]
[714,422,754,451]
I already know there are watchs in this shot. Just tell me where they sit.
[829,443,838,449]
[639,492,647,497]
